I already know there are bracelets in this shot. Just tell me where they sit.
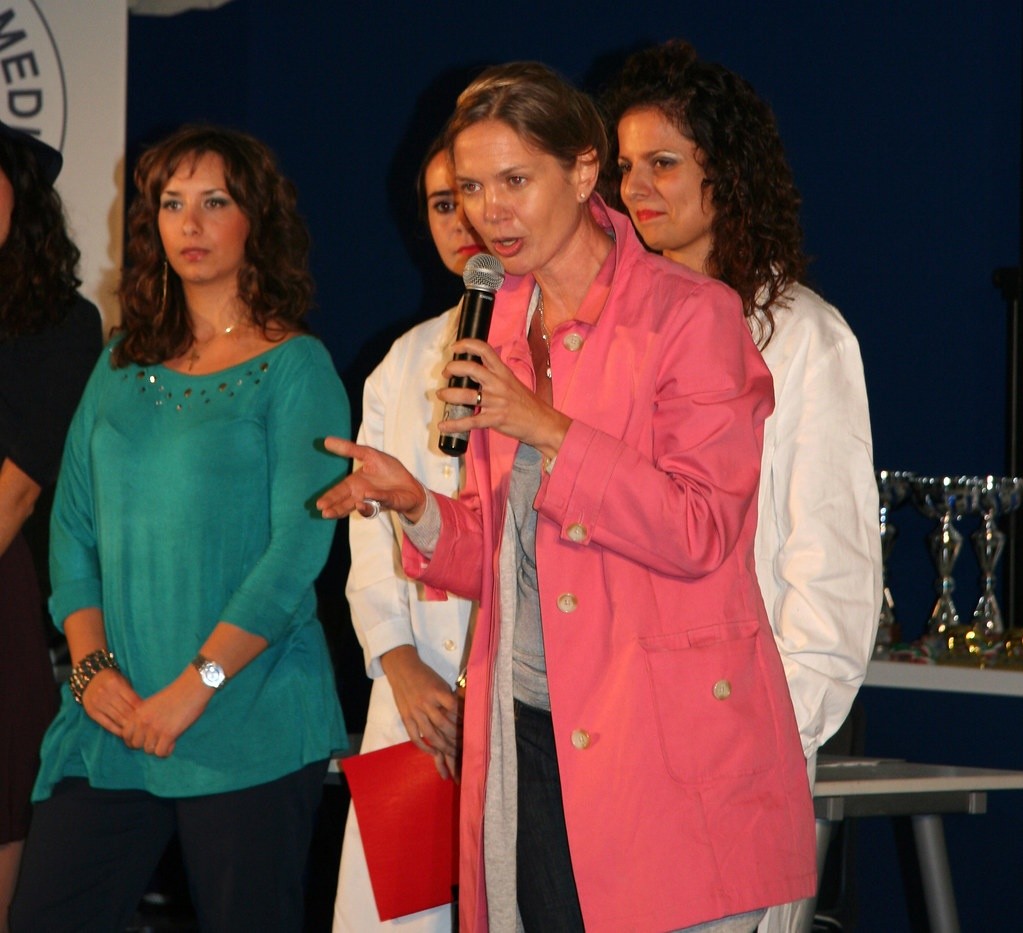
[68,646,123,705]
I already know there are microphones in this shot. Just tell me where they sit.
[437,253,506,456]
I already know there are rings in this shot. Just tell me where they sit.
[362,496,382,518]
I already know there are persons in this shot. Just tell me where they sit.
[611,40,880,776]
[306,61,827,929]
[32,129,350,929]
[323,134,485,933]
[1,123,101,933]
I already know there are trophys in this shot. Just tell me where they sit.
[907,473,975,658]
[957,472,1023,662]
[869,466,918,658]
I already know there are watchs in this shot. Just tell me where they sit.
[190,653,228,691]
[454,668,469,689]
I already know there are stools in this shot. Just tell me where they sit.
[780,753,1023,933]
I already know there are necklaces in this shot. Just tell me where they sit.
[182,304,256,374]
